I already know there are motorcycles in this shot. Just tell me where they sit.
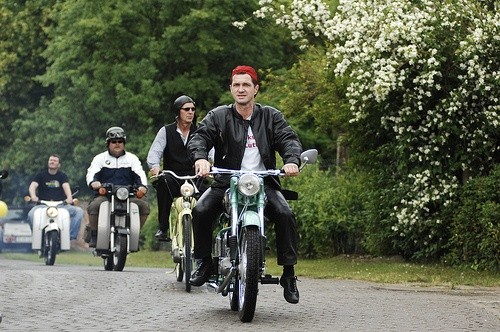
[210,149,318,322]
[94,182,147,271]
[34,199,71,265]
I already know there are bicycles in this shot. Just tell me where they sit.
[149,170,215,293]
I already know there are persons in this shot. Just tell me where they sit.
[145,96,215,239]
[27,155,84,254]
[85,127,151,248]
[186,65,303,304]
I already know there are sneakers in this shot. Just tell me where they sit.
[280,274,299,304]
[189,258,217,286]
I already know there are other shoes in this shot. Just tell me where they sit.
[89,229,97,247]
[155,230,168,240]
[84,226,91,243]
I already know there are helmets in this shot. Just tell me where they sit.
[173,95,195,116]
[105,127,126,147]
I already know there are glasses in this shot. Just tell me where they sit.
[110,140,123,144]
[181,107,197,111]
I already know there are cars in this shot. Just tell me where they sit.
[2,207,34,245]
